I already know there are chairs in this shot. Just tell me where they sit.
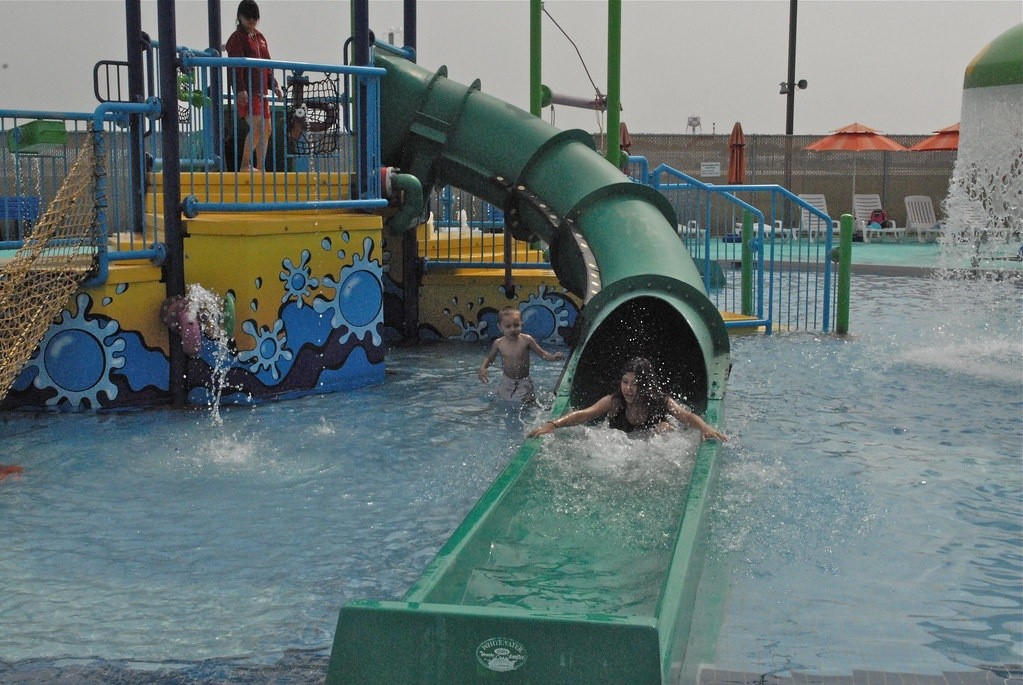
[961,200,1021,244]
[904,195,944,243]
[853,194,905,243]
[798,194,841,243]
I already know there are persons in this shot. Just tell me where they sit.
[476,306,565,401]
[527,357,729,443]
[225,0,280,193]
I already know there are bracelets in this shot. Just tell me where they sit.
[547,421,557,428]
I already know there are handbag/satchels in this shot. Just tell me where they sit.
[871,209,889,228]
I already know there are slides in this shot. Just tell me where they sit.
[321,40,735,685]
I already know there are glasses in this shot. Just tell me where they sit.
[243,13,260,21]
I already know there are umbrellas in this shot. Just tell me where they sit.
[802,121,909,219]
[620,122,632,175]
[909,122,960,152]
[727,122,744,235]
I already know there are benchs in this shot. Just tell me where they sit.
[735,219,790,244]
